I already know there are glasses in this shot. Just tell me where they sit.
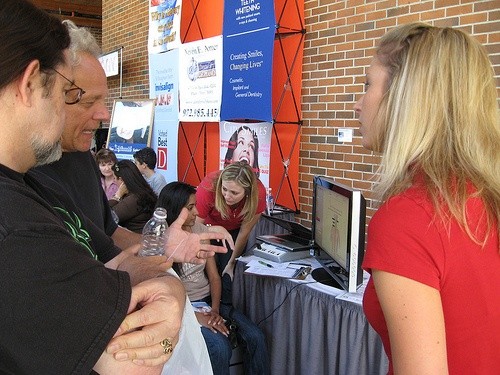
[115,163,120,172]
[53,69,86,104]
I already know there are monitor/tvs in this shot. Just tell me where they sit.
[312,176,367,293]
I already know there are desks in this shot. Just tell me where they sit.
[231,255,389,375]
[247,205,296,251]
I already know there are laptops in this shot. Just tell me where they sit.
[256,213,312,252]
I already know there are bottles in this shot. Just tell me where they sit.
[267,187,273,210]
[139,208,168,257]
